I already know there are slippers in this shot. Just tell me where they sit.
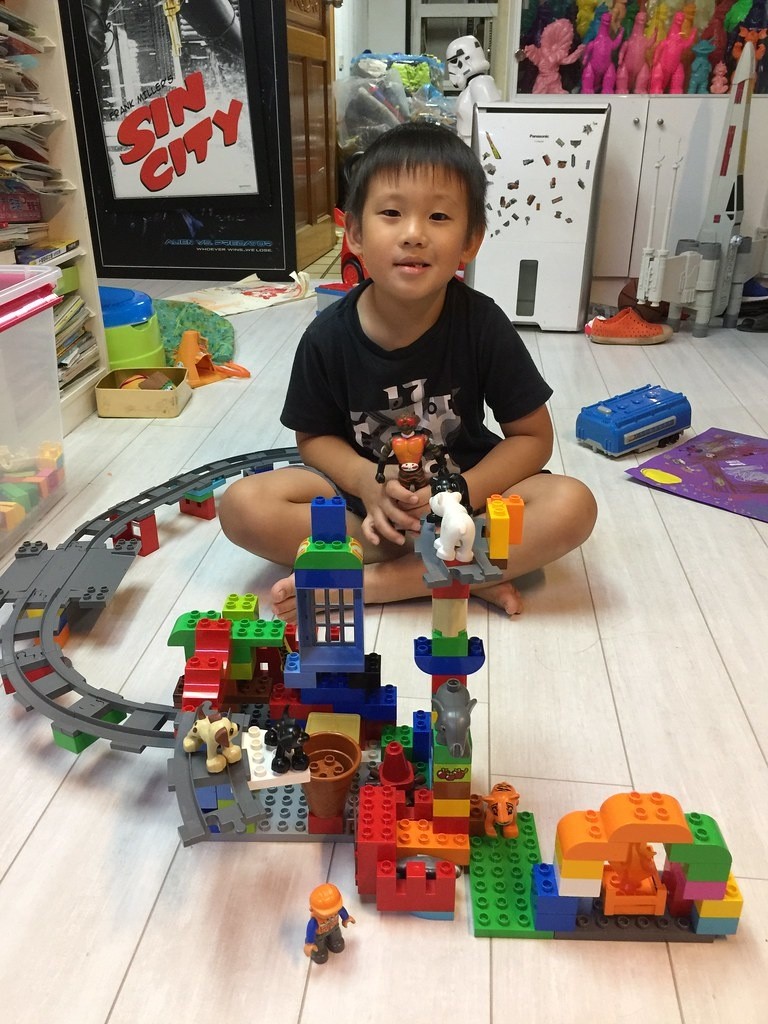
[737,314,768,331]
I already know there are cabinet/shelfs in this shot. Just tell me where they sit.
[508,96,768,310]
[285,0,344,275]
[0,0,110,441]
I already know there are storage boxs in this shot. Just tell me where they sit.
[94,367,193,418]
[0,261,74,558]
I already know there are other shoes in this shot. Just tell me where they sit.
[591,307,673,344]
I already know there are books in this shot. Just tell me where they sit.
[57,264,102,399]
[0,126,80,266]
[0,0,57,118]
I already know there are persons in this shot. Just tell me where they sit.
[446,34,502,148]
[216,122,599,628]
[303,883,356,965]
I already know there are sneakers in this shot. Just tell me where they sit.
[742,278,768,302]
